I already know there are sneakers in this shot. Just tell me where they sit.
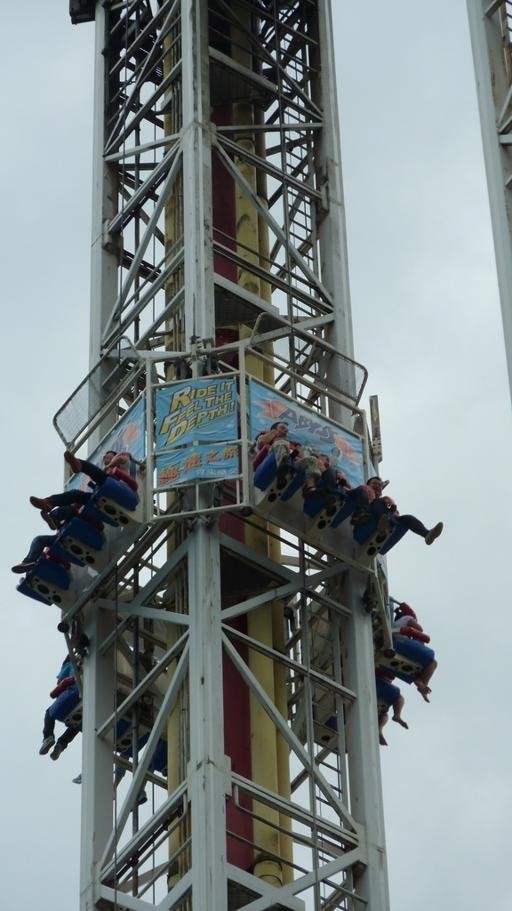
[384,503,397,528]
[130,790,148,813]
[39,736,55,755]
[50,743,64,761]
[302,481,338,506]
[64,450,81,473]
[112,791,116,800]
[72,774,83,785]
[304,465,324,479]
[349,510,371,525]
[275,453,292,480]
[40,510,56,531]
[30,495,53,513]
[425,521,444,545]
[11,561,36,573]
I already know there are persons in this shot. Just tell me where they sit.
[373,600,438,746]
[251,420,443,546]
[11,447,138,589]
[37,652,165,806]
